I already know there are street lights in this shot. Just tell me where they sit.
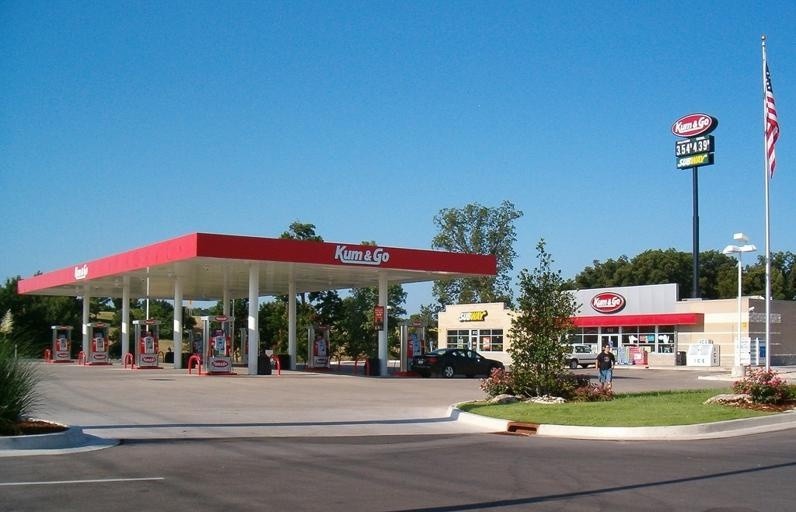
[723,233,757,378]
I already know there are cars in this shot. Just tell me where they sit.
[410,348,505,378]
[550,343,598,368]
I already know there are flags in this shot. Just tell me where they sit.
[765,60,781,181]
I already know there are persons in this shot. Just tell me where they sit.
[595,343,616,392]
